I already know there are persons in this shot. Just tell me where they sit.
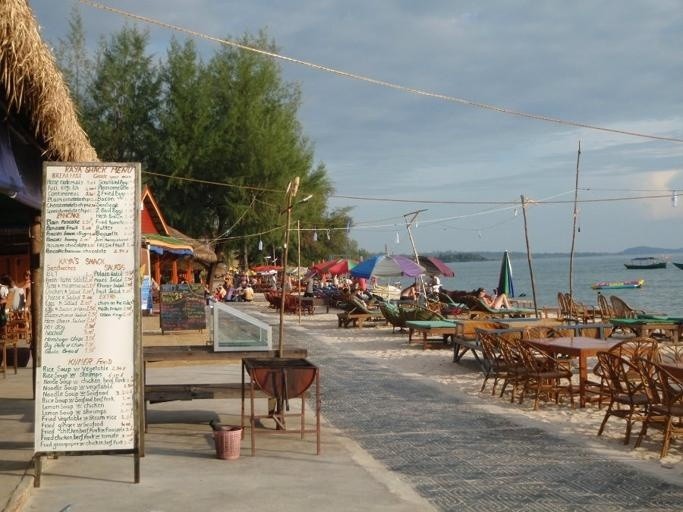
[202,265,377,301]
[401,274,440,299]
[478,286,510,308]
[0,270,35,318]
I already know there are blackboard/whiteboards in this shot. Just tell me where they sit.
[34,161,144,458]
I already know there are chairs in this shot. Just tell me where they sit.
[0,302,32,380]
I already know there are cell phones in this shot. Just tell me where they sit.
[492,288,497,299]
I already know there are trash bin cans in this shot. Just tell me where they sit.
[213,424,243,460]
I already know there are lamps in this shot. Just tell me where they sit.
[313,233,317,241]
[259,241,263,251]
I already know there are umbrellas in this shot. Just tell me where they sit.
[350,255,426,296]
[498,254,514,298]
[413,256,454,277]
[309,259,358,275]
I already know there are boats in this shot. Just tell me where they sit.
[589,279,645,289]
[673,259,683,271]
[624,256,668,270]
